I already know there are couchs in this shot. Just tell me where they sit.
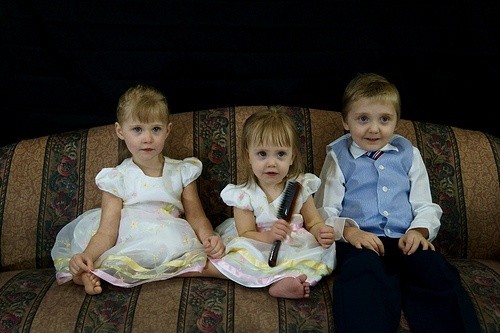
[0,105,500,333]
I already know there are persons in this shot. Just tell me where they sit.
[314,74,482,333]
[50,84,225,295]
[177,109,337,299]
